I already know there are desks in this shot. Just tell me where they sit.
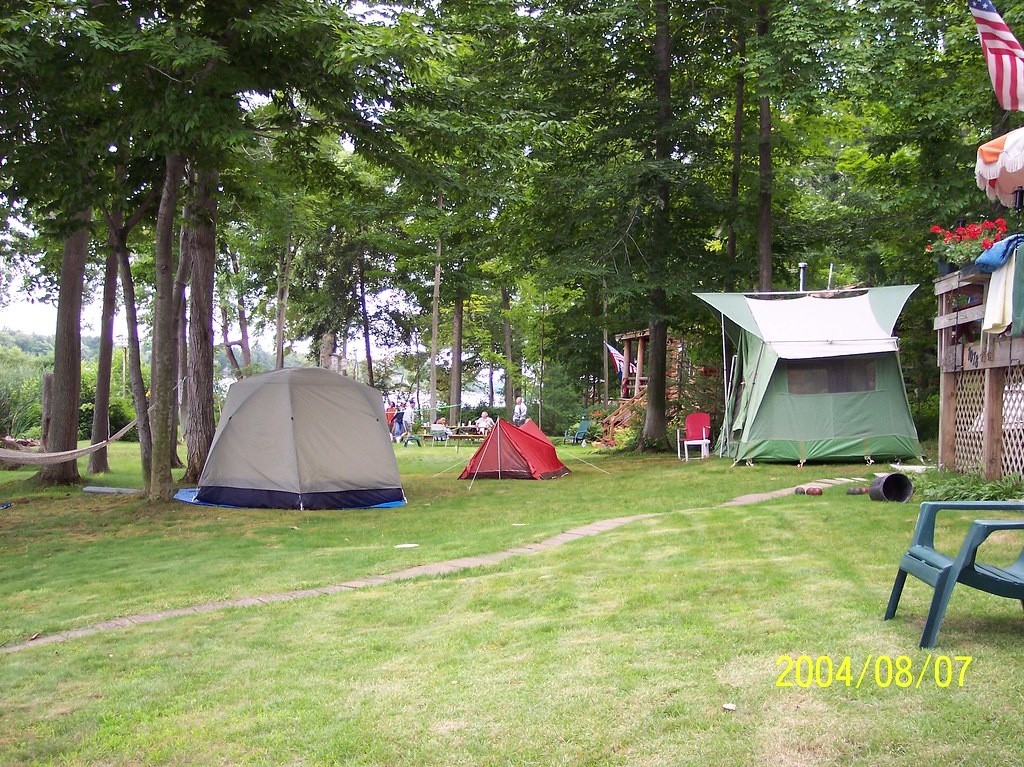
[684,440,710,462]
[421,425,477,447]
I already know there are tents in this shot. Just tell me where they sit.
[194,366,409,510]
[693,283,925,463]
[458,417,571,480]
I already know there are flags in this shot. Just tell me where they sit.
[968,0,1024,112]
[607,345,637,376]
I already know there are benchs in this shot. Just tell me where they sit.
[952,299,1013,345]
[419,434,486,437]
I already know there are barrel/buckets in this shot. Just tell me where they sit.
[869,474,913,503]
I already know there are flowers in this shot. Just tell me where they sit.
[924,218,1008,268]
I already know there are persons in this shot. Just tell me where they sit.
[512,396,527,422]
[434,416,451,441]
[475,411,495,435]
[385,400,414,445]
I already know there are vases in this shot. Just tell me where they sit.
[937,256,958,276]
[957,264,991,282]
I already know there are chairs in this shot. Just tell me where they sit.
[883,501,1024,648]
[563,421,589,445]
[431,430,451,447]
[677,413,711,460]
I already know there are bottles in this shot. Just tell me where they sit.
[581,437,587,448]
[461,416,476,427]
